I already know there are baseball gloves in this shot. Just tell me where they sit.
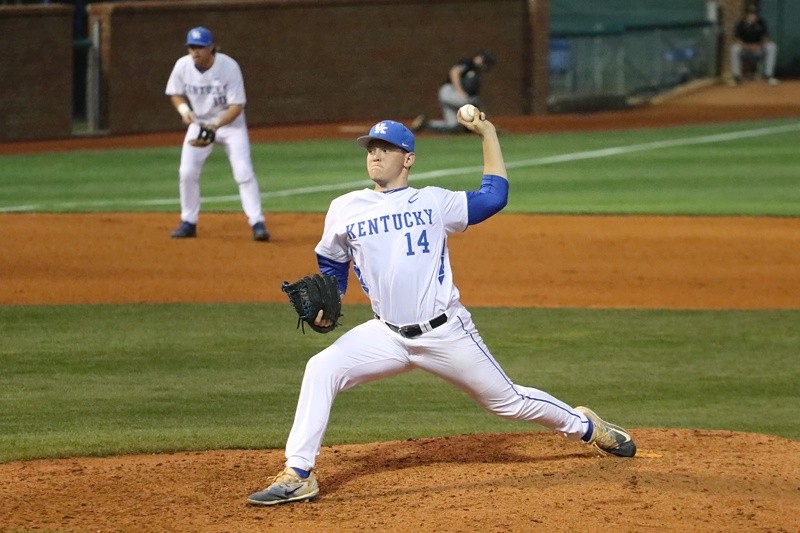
[281,273,342,334]
[188,124,215,146]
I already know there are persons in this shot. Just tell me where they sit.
[409,49,494,136]
[165,27,270,241]
[246,106,637,504]
[722,3,780,87]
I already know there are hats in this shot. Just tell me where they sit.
[185,27,212,46]
[357,120,414,151]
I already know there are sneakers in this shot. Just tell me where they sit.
[248,467,320,506]
[253,224,268,239]
[575,406,635,457]
[172,223,195,238]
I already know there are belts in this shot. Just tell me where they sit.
[375,314,447,337]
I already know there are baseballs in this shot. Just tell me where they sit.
[460,104,476,121]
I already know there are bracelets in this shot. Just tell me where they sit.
[177,102,192,116]
